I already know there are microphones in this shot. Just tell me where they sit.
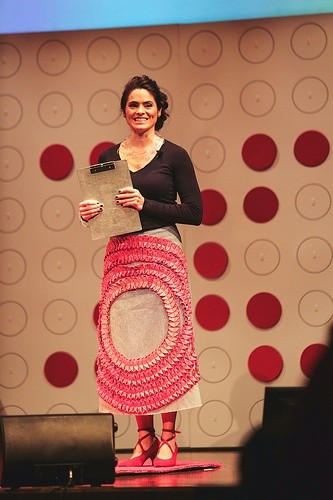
[155,150,163,158]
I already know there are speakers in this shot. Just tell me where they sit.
[0,413,116,486]
[261,385,303,432]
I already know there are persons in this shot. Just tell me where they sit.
[77,75,205,471]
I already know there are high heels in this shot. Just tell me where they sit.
[117,428,158,466]
[153,429,178,467]
